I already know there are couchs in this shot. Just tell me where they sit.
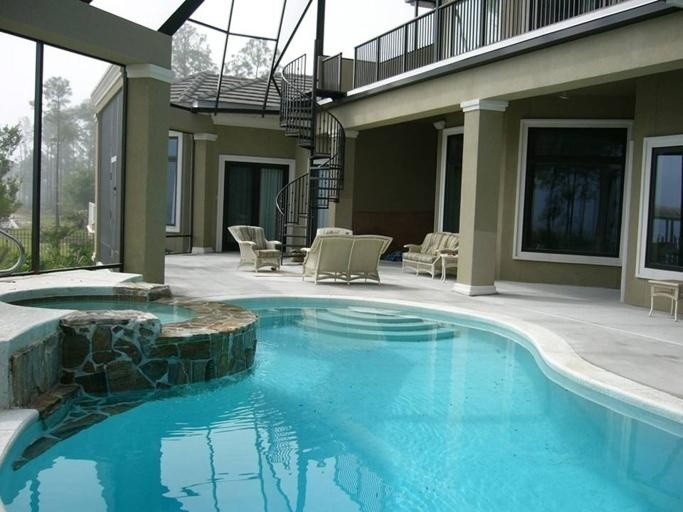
[400,232,459,282]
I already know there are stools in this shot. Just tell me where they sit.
[646,280,682,322]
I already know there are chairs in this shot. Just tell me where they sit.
[226,224,393,287]
